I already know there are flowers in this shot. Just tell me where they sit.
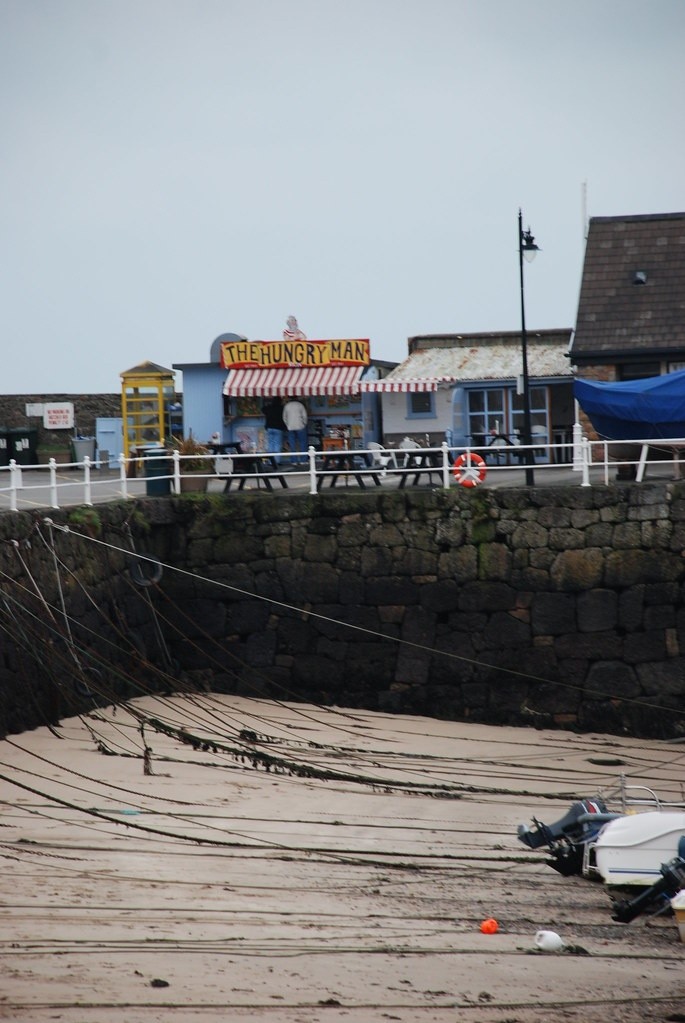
[166,427,215,472]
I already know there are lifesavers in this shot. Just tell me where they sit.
[454,453,486,487]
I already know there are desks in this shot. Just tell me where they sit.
[322,438,350,461]
[464,433,538,465]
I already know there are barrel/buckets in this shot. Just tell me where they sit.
[145,449,170,496]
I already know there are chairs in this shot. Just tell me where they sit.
[398,452,443,490]
[206,442,288,494]
[308,444,381,490]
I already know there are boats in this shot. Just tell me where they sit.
[518,770,685,942]
[573,368,685,461]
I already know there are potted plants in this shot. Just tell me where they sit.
[35,443,72,471]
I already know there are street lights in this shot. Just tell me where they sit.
[517,208,539,486]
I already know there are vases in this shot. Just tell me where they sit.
[179,470,211,493]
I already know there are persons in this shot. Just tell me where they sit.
[261,396,285,466]
[282,396,309,465]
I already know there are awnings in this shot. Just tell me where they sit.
[223,366,364,397]
[356,378,457,392]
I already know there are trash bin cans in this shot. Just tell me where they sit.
[144,449,171,496]
[0,426,10,471]
[10,427,37,472]
[72,436,96,469]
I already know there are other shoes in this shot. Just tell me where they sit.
[290,462,298,466]
[300,461,309,465]
